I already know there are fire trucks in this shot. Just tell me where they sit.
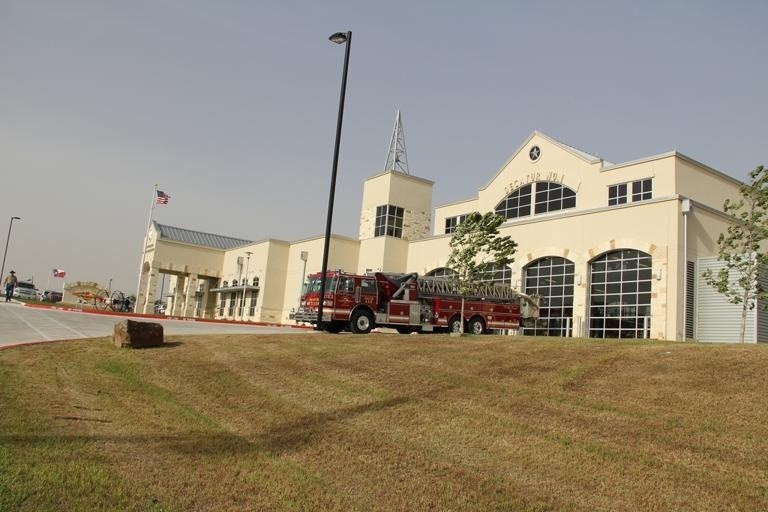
[292,269,521,335]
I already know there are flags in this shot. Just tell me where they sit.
[53,267,66,278]
[156,191,172,205]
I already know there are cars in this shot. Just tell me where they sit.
[13,281,63,303]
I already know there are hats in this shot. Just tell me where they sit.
[10,270,15,273]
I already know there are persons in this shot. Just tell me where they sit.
[125,297,130,311]
[3,270,18,302]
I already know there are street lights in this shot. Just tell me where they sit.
[0,217,21,285]
[315,31,351,331]
